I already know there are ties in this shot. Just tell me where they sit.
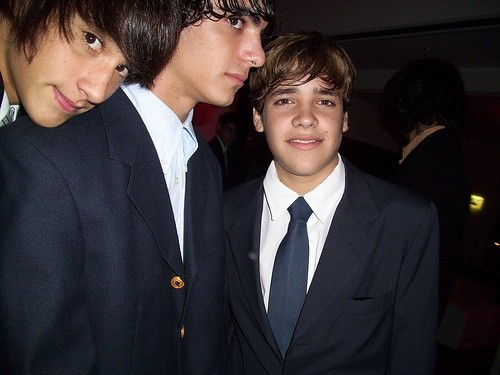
[267,196,314,362]
[0,107,16,126]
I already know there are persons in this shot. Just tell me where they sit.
[1,0,276,375]
[0,0,183,129]
[224,31,440,374]
[207,111,247,191]
[385,60,498,334]
